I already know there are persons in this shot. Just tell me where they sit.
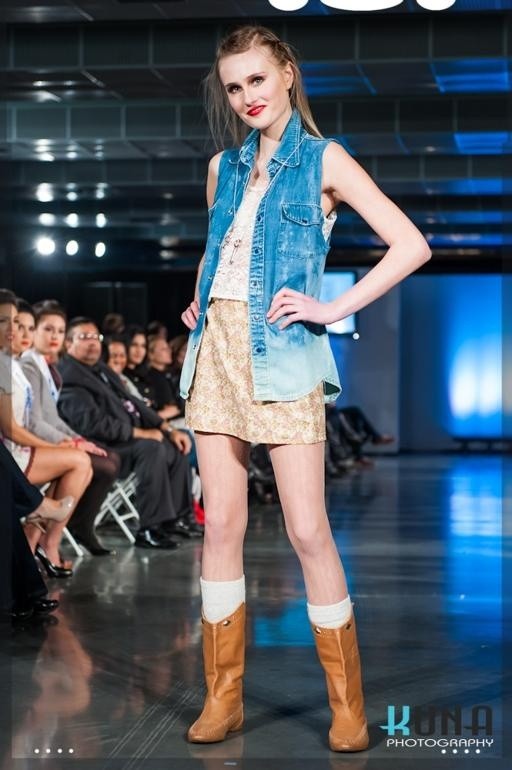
[0,284,398,630]
[178,21,433,753]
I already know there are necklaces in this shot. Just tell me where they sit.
[229,132,311,267]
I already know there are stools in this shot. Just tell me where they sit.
[35,467,141,559]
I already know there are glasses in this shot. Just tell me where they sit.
[76,332,103,341]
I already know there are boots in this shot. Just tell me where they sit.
[310,610,371,753]
[187,603,246,745]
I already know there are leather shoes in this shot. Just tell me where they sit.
[325,457,374,479]
[11,600,58,616]
[133,516,203,549]
[36,547,72,579]
[75,535,116,557]
[372,434,395,445]
